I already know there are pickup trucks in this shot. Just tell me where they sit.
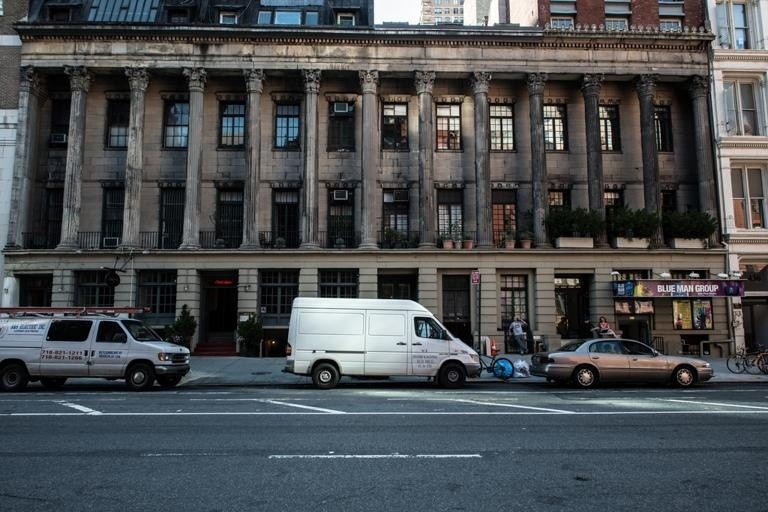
[0,314,191,394]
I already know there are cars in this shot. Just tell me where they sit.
[529,338,715,389]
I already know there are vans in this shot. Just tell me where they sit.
[281,297,483,390]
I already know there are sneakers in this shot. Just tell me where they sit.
[520,349,528,355]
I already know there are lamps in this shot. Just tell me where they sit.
[609,269,728,280]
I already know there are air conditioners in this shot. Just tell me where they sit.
[333,103,349,114]
[104,237,121,248]
[51,132,67,144]
[334,190,349,200]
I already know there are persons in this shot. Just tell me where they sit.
[508,316,530,355]
[598,316,620,337]
[580,317,600,338]
[418,320,433,339]
[557,316,568,334]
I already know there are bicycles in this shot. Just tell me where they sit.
[473,348,515,380]
[726,343,768,376]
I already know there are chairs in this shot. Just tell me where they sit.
[604,344,625,354]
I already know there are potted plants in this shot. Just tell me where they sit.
[213,239,224,248]
[439,232,453,250]
[504,232,516,249]
[452,219,464,249]
[518,230,534,250]
[463,234,474,250]
[541,209,604,249]
[237,313,262,357]
[661,208,718,250]
[173,306,196,352]
[274,238,286,248]
[605,202,660,249]
[334,238,346,249]
